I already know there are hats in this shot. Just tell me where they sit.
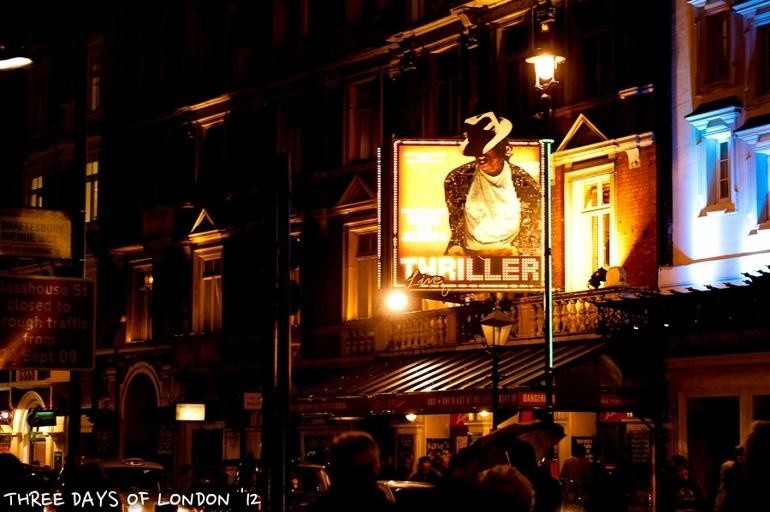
[460,111,514,157]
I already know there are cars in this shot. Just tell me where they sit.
[289,463,443,512]
[62,458,196,512]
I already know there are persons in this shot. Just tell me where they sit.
[444,111,542,283]
[308,419,770,512]
[32,455,72,488]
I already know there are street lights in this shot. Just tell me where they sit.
[479,307,519,437]
[524,17,576,415]
